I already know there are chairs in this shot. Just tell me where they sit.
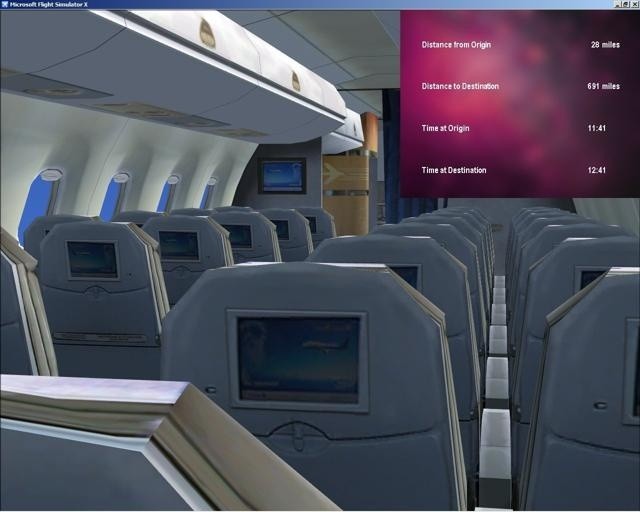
[0,204,640,512]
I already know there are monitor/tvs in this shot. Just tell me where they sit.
[227,308,367,416]
[388,263,421,291]
[572,265,607,294]
[257,158,305,195]
[269,217,290,242]
[157,228,200,263]
[304,215,318,235]
[133,221,145,232]
[623,318,640,423]
[63,238,119,282]
[43,227,51,236]
[216,222,254,253]
